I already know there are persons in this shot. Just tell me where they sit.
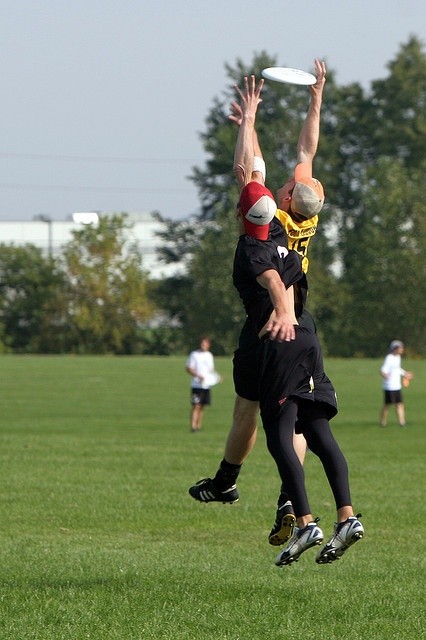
[226,101,365,567]
[185,337,221,431]
[378,340,415,427]
[189,59,330,546]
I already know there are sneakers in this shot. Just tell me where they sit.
[268,501,297,546]
[189,479,239,505]
[275,517,324,566]
[316,513,364,564]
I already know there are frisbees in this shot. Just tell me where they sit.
[203,372,220,386]
[261,67,317,86]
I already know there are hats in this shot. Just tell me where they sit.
[291,161,324,222]
[240,182,277,240]
